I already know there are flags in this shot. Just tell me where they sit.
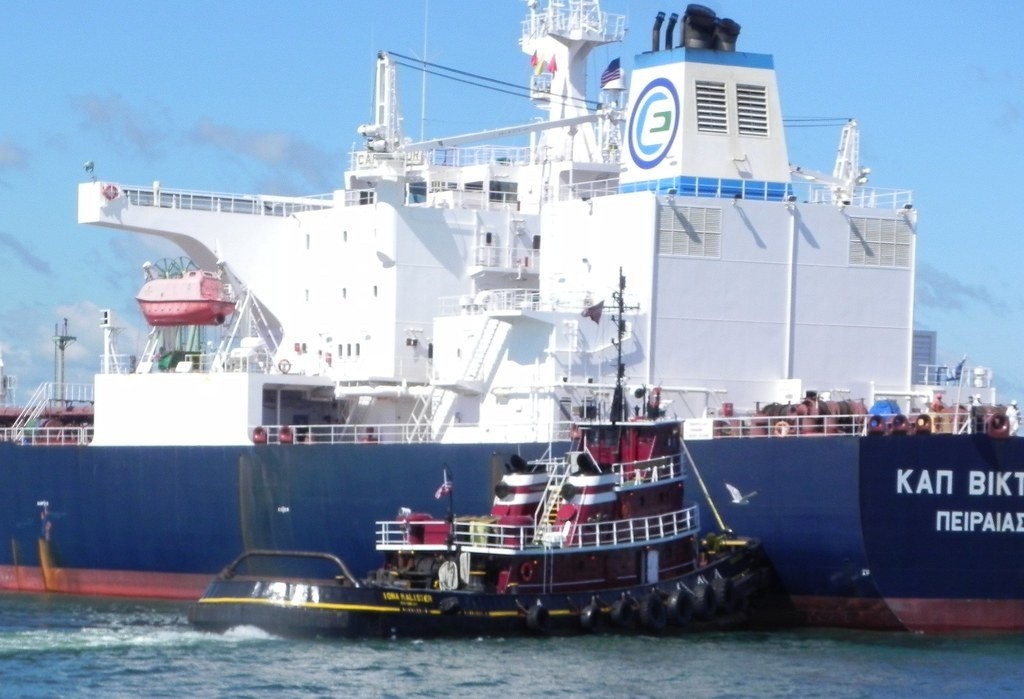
[942,358,966,381]
[531,47,557,79]
[434,468,453,501]
[600,57,621,88]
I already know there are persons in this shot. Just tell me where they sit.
[1005,401,1021,436]
[967,393,982,412]
[931,394,948,433]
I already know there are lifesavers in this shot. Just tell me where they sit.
[526,577,735,635]
[775,421,790,436]
[521,563,534,582]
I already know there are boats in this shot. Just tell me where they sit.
[0,0,1022,636]
[182,265,794,641]
